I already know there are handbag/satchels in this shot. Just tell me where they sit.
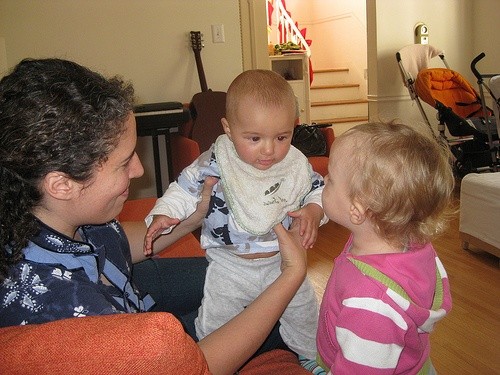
[291,123,332,156]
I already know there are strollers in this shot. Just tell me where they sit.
[395,44,500,193]
[470,52,500,172]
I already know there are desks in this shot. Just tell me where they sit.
[134,101,184,198]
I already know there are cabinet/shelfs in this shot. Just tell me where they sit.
[270,54,311,124]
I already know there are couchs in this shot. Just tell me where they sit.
[171,104,335,183]
[0,198,313,375]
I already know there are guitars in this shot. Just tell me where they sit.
[188,31,226,155]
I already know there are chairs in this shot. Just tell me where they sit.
[459,173,500,259]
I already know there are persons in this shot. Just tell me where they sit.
[0,58,307,375]
[143,69,329,375]
[316,118,455,375]
